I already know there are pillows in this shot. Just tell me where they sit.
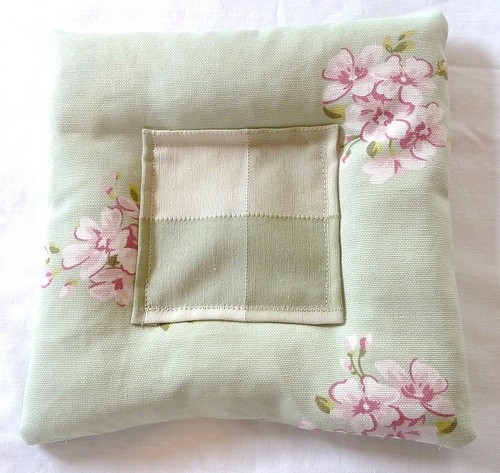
[18,7,478,451]
